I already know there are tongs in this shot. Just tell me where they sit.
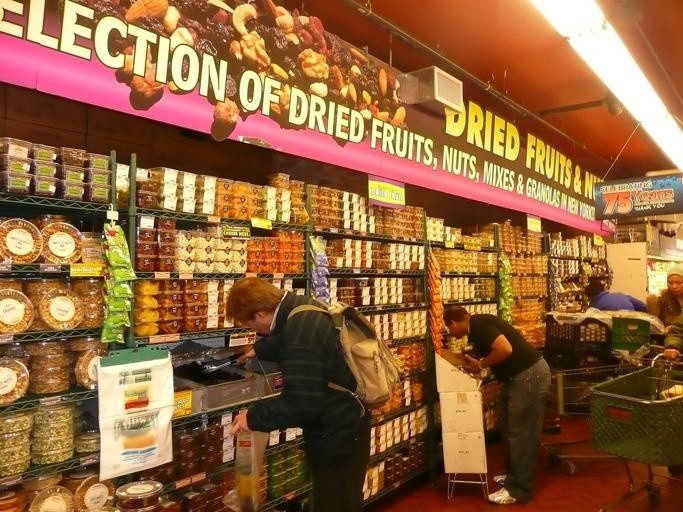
[202,358,242,375]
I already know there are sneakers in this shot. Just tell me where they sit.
[488,486,516,505]
[493,474,506,486]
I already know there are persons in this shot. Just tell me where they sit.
[443,305,552,504]
[226,276,374,512]
[585,281,649,310]
[657,267,683,362]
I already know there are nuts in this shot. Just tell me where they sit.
[57,0,410,144]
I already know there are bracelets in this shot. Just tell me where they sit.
[479,358,487,371]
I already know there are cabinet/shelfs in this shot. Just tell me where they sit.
[1,137,613,512]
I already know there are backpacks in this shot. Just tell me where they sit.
[328,297,402,408]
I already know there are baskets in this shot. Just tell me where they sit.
[539,309,651,372]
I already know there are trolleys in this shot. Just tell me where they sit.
[546,312,683,506]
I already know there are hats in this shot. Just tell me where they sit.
[666,265,682,278]
